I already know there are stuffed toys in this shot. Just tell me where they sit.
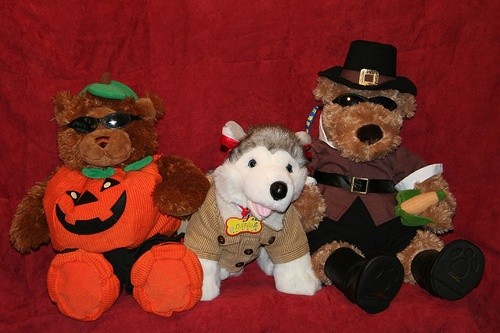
[8,71,211,323]
[291,39,486,314]
[182,120,322,302]
